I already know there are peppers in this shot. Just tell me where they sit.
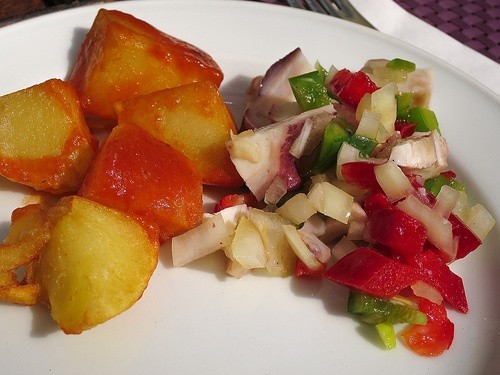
[295,69,483,357]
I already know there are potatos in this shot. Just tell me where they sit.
[1,8,256,336]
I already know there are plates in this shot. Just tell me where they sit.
[0,2,499,375]
